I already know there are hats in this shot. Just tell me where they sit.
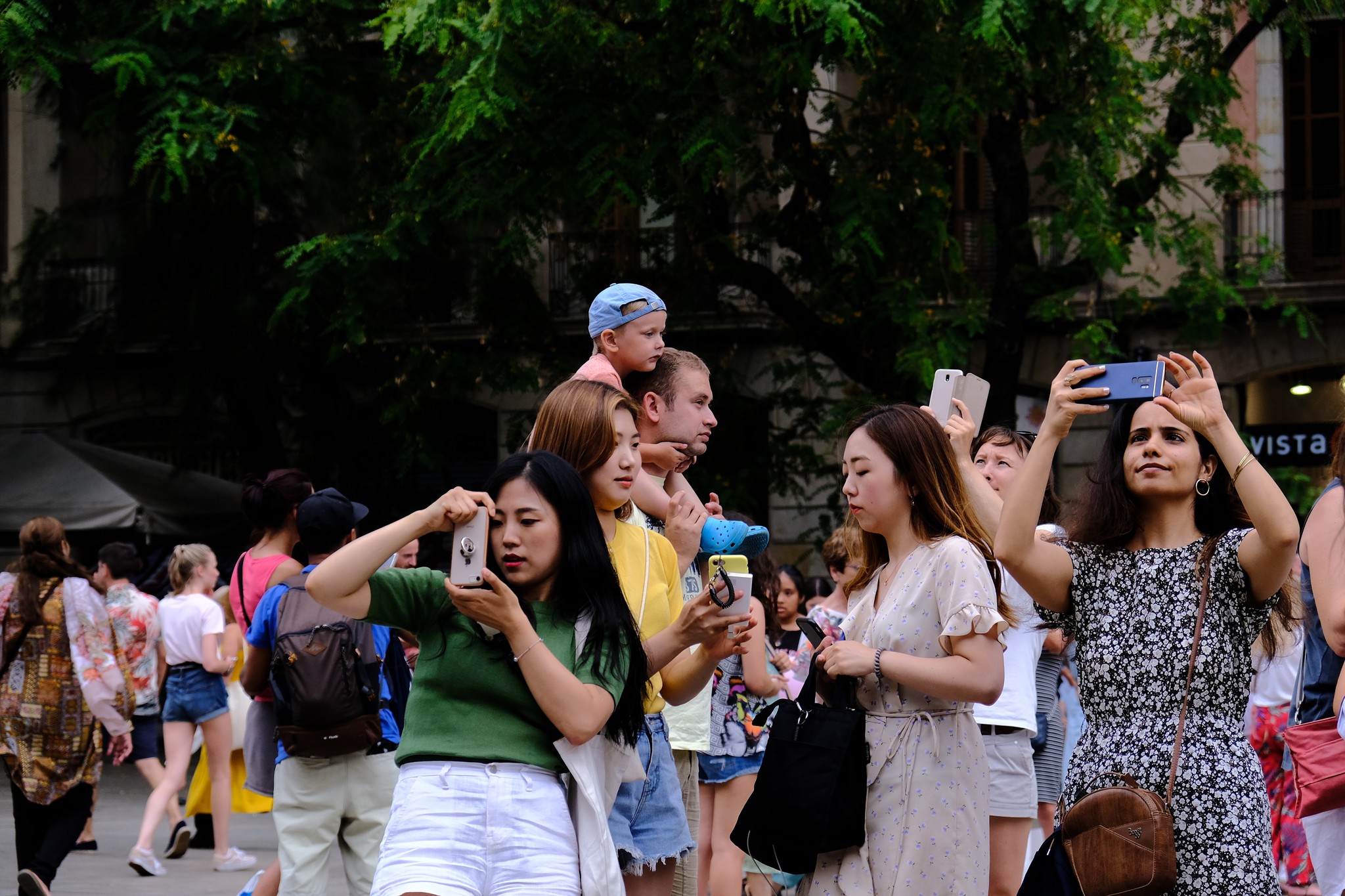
[588,282,666,337]
[295,487,370,541]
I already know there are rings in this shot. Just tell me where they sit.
[1167,388,1176,400]
[1064,372,1076,386]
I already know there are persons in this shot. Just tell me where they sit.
[0,283,1345,896]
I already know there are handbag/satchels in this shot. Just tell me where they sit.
[1061,773,1175,896]
[1030,712,1048,754]
[729,619,866,876]
[1282,716,1345,820]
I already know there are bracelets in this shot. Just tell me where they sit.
[874,646,886,679]
[1230,451,1256,488]
[513,636,544,661]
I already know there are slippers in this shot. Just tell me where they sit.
[701,517,770,560]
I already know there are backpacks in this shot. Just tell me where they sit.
[270,572,382,759]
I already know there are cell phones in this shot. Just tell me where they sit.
[708,555,749,588]
[929,368,963,429]
[1070,360,1167,405]
[377,553,398,571]
[796,617,834,649]
[450,507,490,587]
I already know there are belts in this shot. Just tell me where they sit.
[977,723,1024,735]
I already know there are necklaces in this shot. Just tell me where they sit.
[884,538,923,586]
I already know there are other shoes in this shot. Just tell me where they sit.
[212,846,256,870]
[189,812,214,850]
[128,846,167,876]
[163,820,191,859]
[71,839,98,853]
[17,869,50,896]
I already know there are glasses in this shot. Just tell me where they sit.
[1014,430,1037,440]
